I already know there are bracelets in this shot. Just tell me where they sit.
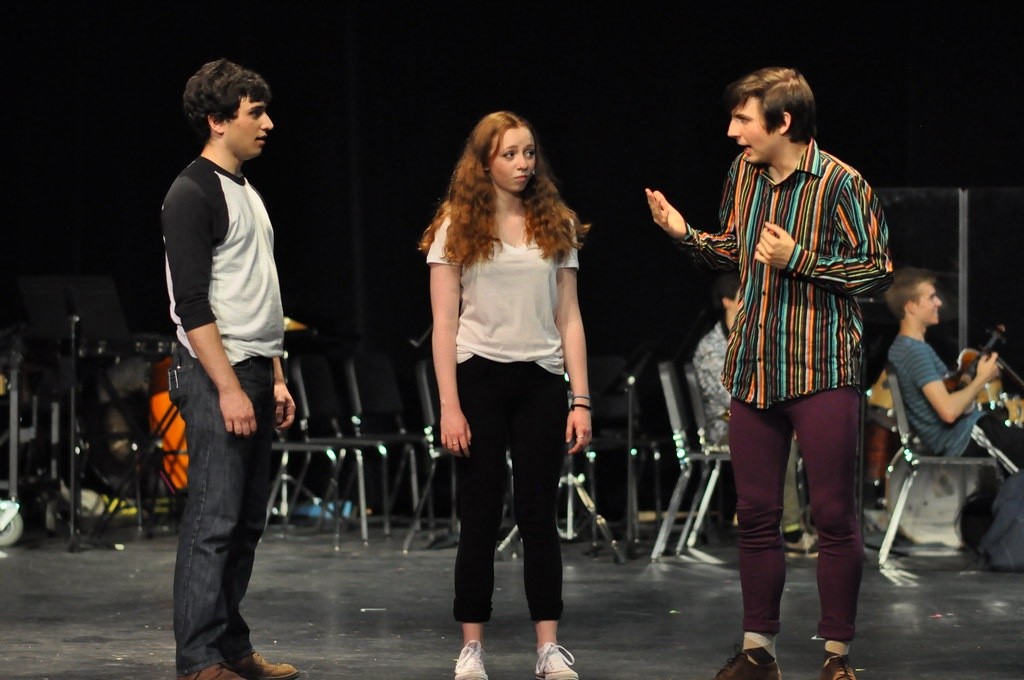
[573,395,591,400]
[571,403,592,411]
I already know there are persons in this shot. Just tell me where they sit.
[689,271,823,560]
[415,109,594,680]
[644,68,893,679]
[881,266,1023,575]
[160,59,297,680]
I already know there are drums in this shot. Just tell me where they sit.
[865,369,898,433]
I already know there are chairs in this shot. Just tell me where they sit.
[565,353,731,563]
[877,363,1004,569]
[264,350,470,553]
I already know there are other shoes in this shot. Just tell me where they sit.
[820,654,856,680]
[177,653,300,680]
[710,644,782,680]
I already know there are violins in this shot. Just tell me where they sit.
[937,322,1007,414]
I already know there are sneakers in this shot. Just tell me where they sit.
[785,533,819,558]
[453,640,488,680]
[534,642,579,680]
